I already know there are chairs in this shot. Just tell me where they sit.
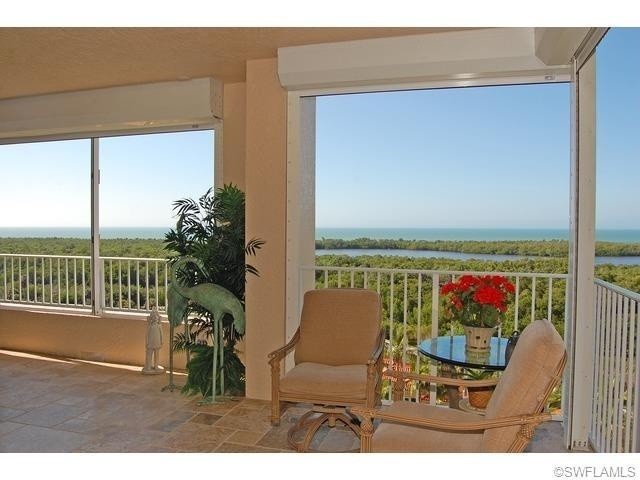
[267,288,388,427]
[350,316,568,453]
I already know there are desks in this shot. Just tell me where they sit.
[418,334,508,411]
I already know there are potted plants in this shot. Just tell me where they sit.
[463,368,498,407]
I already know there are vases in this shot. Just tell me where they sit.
[462,322,499,354]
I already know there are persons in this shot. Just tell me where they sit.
[145,311,164,370]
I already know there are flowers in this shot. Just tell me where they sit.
[440,271,518,330]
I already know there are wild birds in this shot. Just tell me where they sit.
[159,255,246,408]
